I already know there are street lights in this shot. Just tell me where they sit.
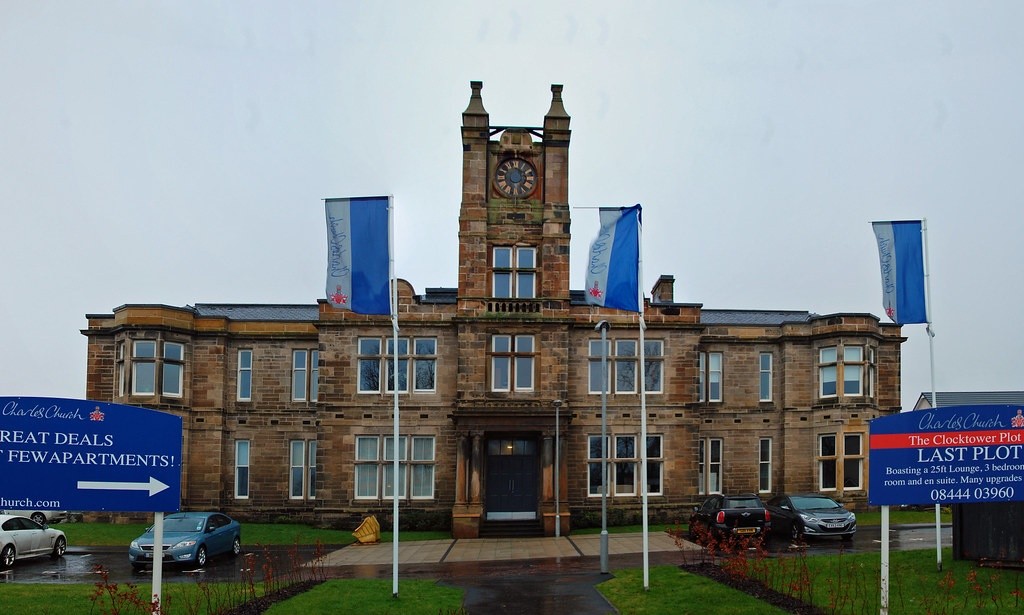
[593,320,610,573]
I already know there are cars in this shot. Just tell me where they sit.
[770,494,858,542]
[0,510,67,524]
[689,492,771,543]
[0,513,68,566]
[129,512,242,570]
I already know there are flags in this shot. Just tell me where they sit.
[584,206,644,311]
[869,221,926,325]
[325,196,390,317]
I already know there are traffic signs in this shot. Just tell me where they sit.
[0,395,181,511]
